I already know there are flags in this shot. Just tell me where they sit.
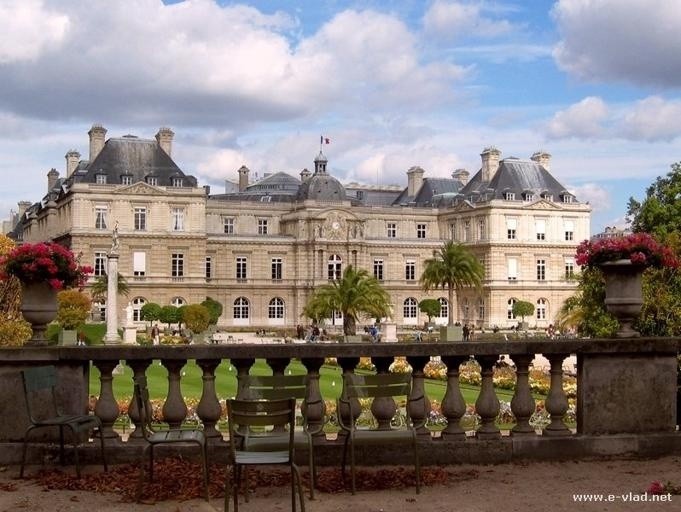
[321,135,329,144]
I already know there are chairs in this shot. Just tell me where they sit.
[224,399,305,512]
[236,375,324,502]
[338,370,428,495]
[20,364,108,480]
[131,375,210,505]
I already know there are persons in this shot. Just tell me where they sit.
[79,328,86,346]
[463,324,469,341]
[151,324,159,345]
[470,325,475,340]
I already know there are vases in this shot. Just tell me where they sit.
[600,259,643,338]
[17,275,58,346]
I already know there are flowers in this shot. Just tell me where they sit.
[638,479,681,496]
[0,241,93,298]
[574,232,681,272]
